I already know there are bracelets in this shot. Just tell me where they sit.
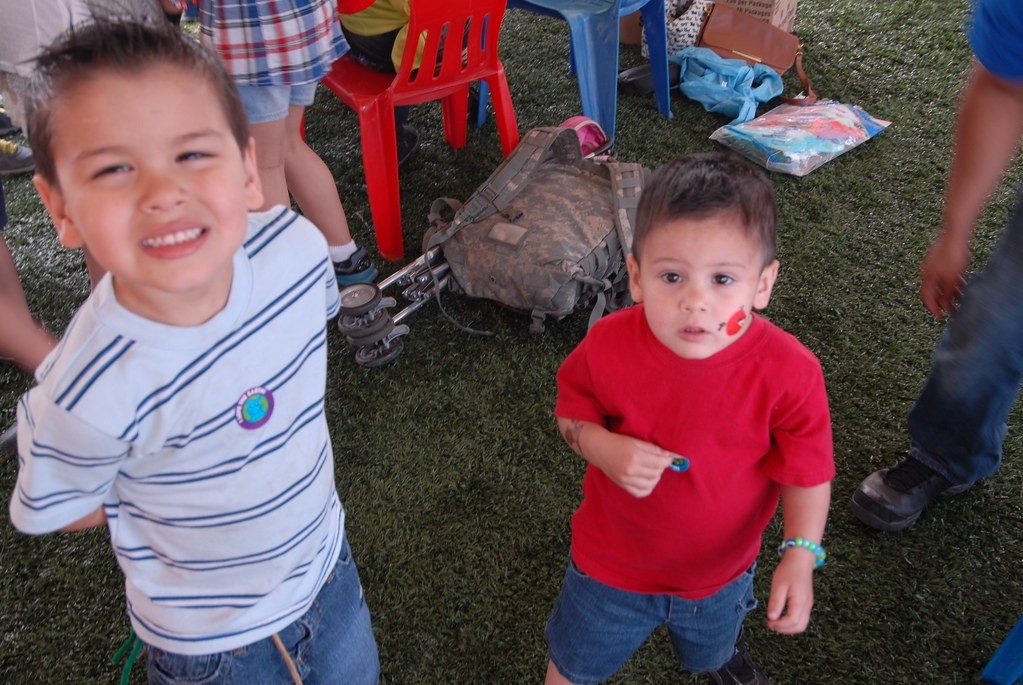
[778,537,826,570]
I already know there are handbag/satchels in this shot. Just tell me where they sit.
[640,0,816,124]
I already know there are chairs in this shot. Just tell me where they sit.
[299,0,519,263]
[474,0,673,147]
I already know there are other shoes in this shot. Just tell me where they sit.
[330,248,379,286]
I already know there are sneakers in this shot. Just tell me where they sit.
[0,137,36,175]
[0,106,22,135]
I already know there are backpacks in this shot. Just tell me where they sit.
[423,125,650,321]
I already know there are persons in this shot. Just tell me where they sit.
[8,21,380,685]
[544,152,835,685]
[0,0,479,375]
[849,0,1023,533]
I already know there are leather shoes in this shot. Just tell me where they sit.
[851,453,971,529]
